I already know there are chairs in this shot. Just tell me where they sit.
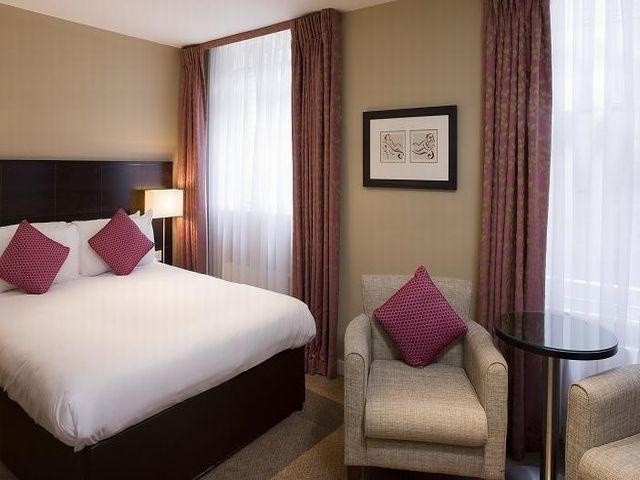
[564,364,640,480]
[341,273,508,479]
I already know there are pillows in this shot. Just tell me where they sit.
[372,265,468,369]
[0,208,158,293]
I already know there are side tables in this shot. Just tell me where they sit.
[492,310,617,480]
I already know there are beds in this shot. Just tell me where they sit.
[0,159,316,478]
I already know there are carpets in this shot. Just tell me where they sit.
[0,387,345,479]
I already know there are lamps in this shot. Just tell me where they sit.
[144,186,184,263]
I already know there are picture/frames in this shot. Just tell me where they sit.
[361,105,459,192]
